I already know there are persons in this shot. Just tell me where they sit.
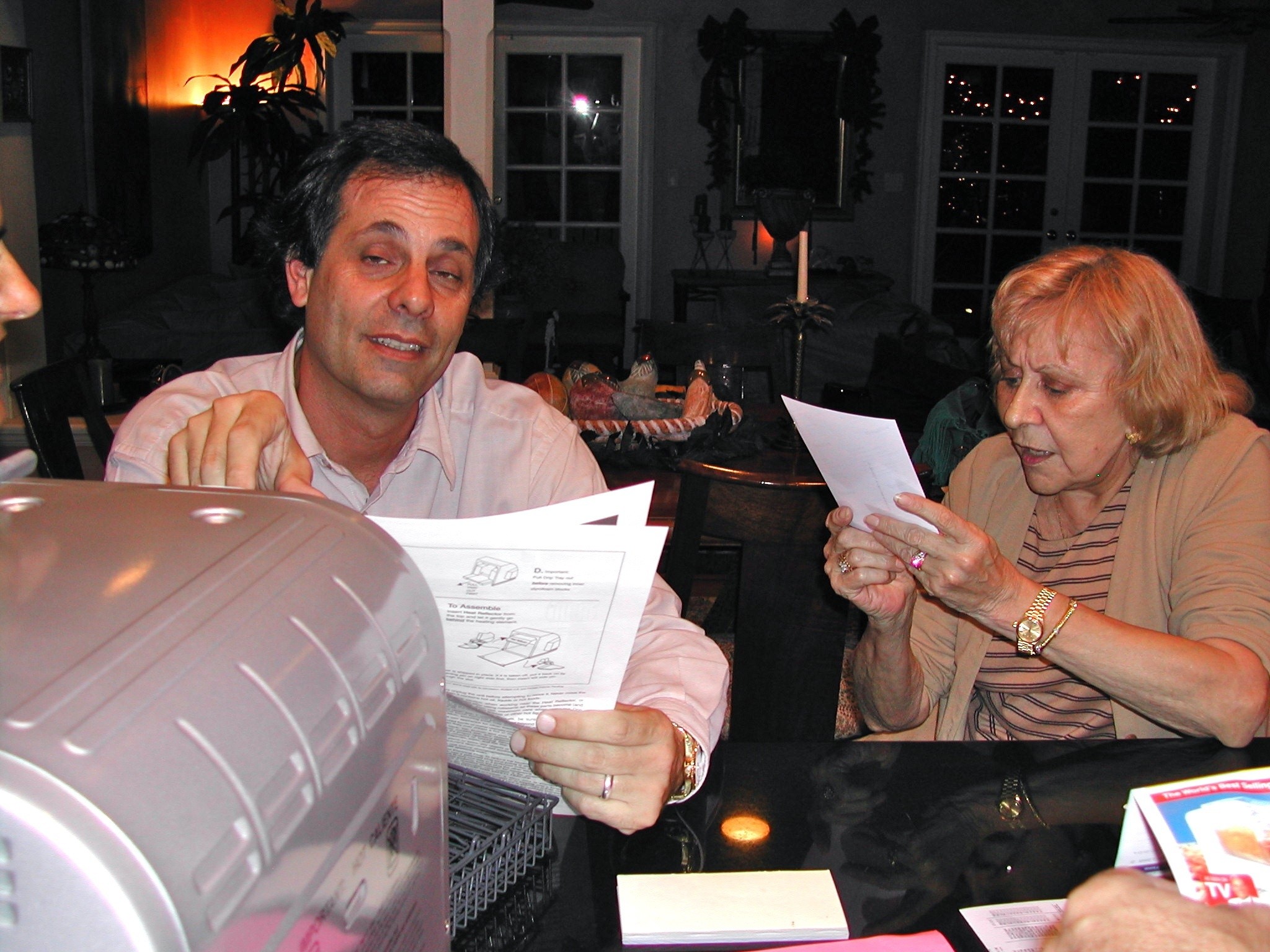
[1040,867,1270,951]
[104,119,730,836]
[0,199,42,348]
[803,741,1253,938]
[821,242,1270,749]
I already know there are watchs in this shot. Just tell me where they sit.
[998,775,1027,832]
[670,720,703,802]
[1012,586,1057,656]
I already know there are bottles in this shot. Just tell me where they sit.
[685,359,711,391]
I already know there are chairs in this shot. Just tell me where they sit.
[663,458,934,741]
[9,353,115,479]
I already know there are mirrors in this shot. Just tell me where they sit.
[728,29,856,221]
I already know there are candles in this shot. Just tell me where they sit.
[797,231,808,302]
[696,194,710,232]
[720,178,734,230]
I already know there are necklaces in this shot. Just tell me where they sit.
[1054,496,1072,551]
[903,810,913,824]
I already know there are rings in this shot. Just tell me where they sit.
[837,551,853,574]
[909,550,927,570]
[600,773,614,799]
[823,782,836,802]
[888,854,899,869]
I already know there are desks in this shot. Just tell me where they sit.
[450,737,1270,952]
[671,268,894,323]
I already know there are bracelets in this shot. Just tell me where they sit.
[1019,779,1051,832]
[1035,597,1078,656]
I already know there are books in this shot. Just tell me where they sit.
[618,869,849,944]
